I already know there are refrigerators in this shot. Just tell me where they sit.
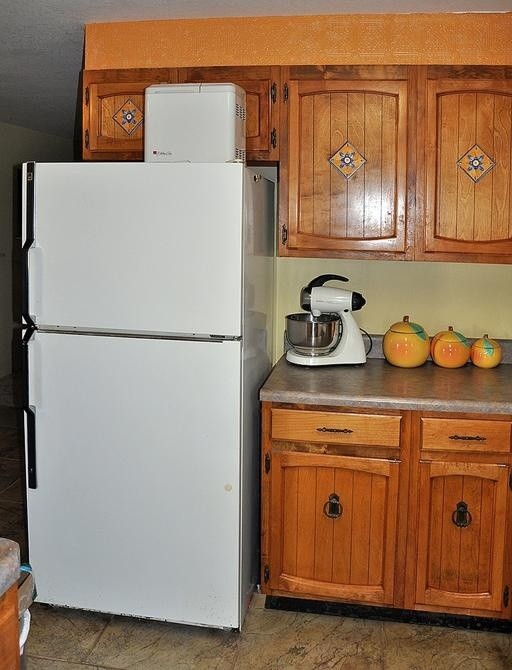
[10,158,280,633]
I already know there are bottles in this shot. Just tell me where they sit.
[471,334,502,369]
[429,323,472,370]
[380,314,430,369]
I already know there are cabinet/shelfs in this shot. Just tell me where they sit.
[80,64,285,164]
[260,402,512,620]
[282,66,510,265]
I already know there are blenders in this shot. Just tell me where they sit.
[286,271,369,368]
